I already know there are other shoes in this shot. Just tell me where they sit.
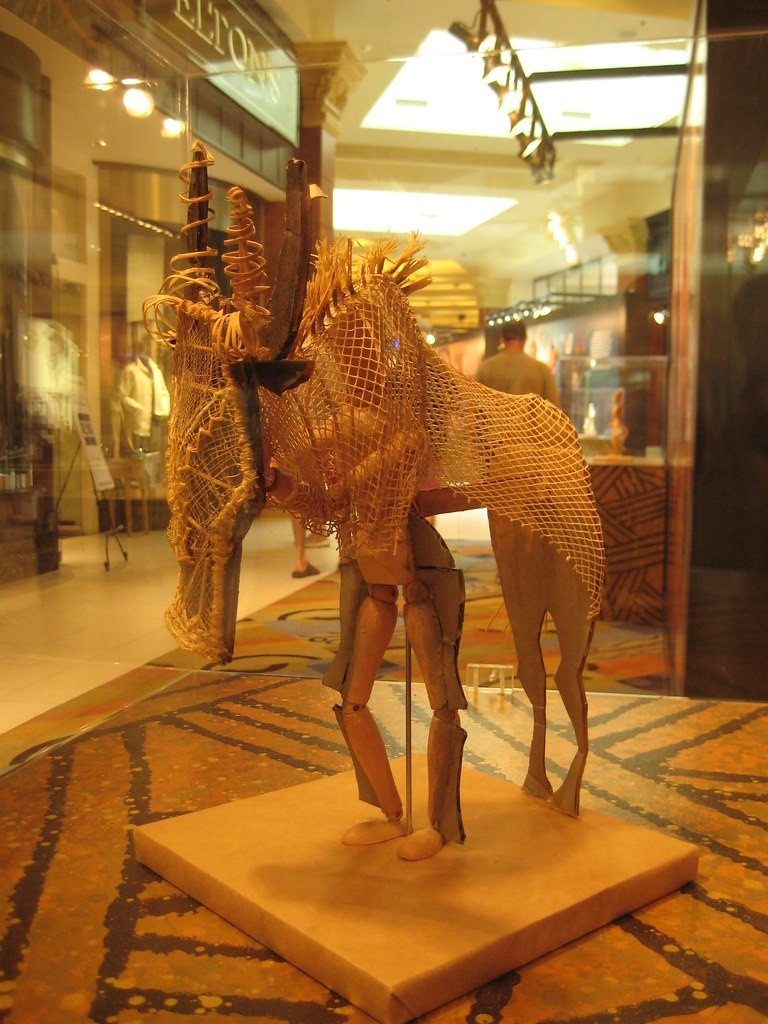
[303,533,330,547]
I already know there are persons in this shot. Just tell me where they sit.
[119,352,171,454]
[473,320,559,406]
[692,273,768,691]
[304,516,331,547]
[292,513,321,577]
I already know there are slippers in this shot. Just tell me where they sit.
[293,562,319,577]
